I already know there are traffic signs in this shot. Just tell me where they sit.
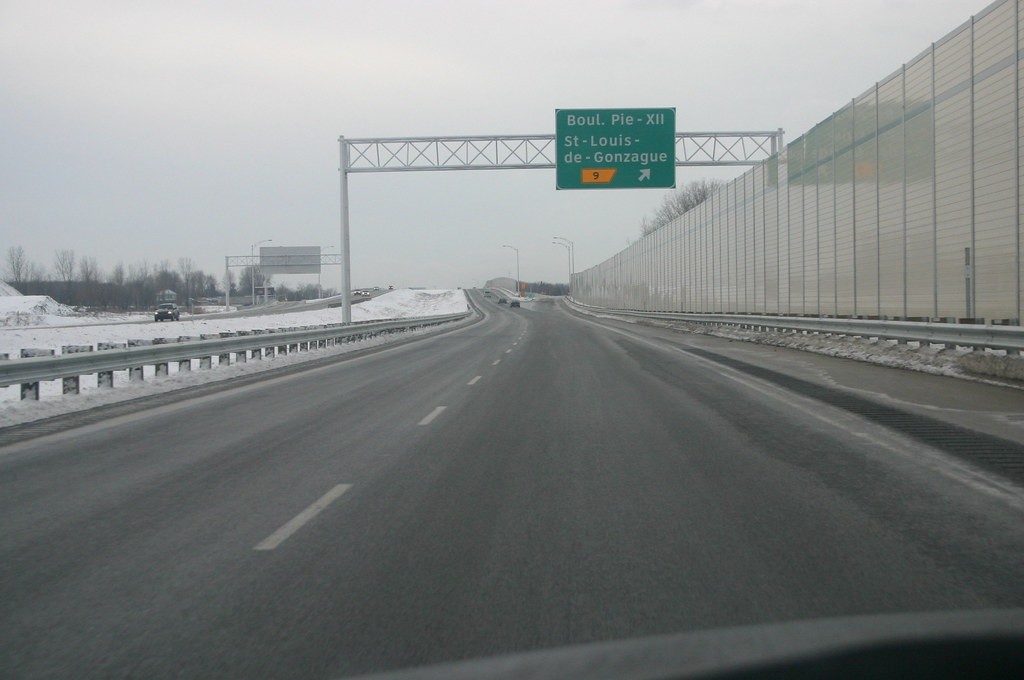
[557,108,677,189]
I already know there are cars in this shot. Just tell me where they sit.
[484,291,491,297]
[354,289,361,294]
[362,291,370,297]
[389,286,393,289]
[509,299,521,308]
[373,286,379,291]
[498,297,507,304]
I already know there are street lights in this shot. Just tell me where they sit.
[318,245,335,296]
[502,244,519,295]
[251,237,271,306]
[552,240,571,297]
[554,235,577,303]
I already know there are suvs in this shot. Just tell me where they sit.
[155,302,181,323]
